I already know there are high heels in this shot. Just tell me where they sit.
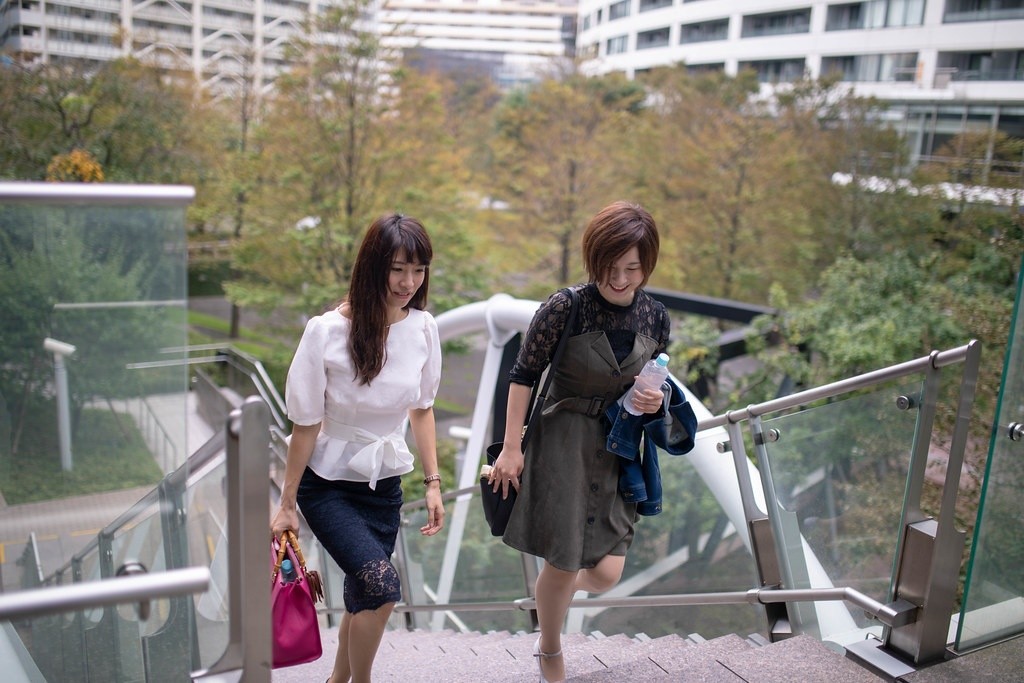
[533,635,565,683]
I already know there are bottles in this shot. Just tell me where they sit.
[623,353,669,416]
[281,560,296,585]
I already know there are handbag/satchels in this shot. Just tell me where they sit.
[480,442,525,537]
[271,531,323,669]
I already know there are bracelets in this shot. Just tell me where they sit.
[424,474,440,485]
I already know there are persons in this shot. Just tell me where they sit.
[272,211,445,683]
[485,200,670,683]
